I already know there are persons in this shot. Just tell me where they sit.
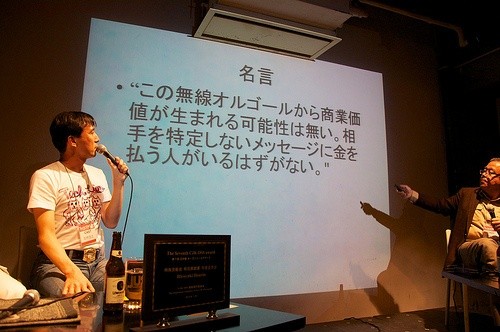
[27,112,129,297]
[395,158,500,277]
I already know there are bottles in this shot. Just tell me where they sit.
[102,232,125,323]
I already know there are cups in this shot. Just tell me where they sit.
[126,270,143,300]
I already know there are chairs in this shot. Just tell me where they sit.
[444,229,494,332]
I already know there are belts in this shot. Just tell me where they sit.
[65,249,99,263]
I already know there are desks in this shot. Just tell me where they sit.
[444,271,500,332]
[0,297,306,332]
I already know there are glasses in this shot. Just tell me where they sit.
[479,169,500,178]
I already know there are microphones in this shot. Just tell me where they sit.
[486,204,500,237]
[0,288,41,320]
[96,144,130,176]
